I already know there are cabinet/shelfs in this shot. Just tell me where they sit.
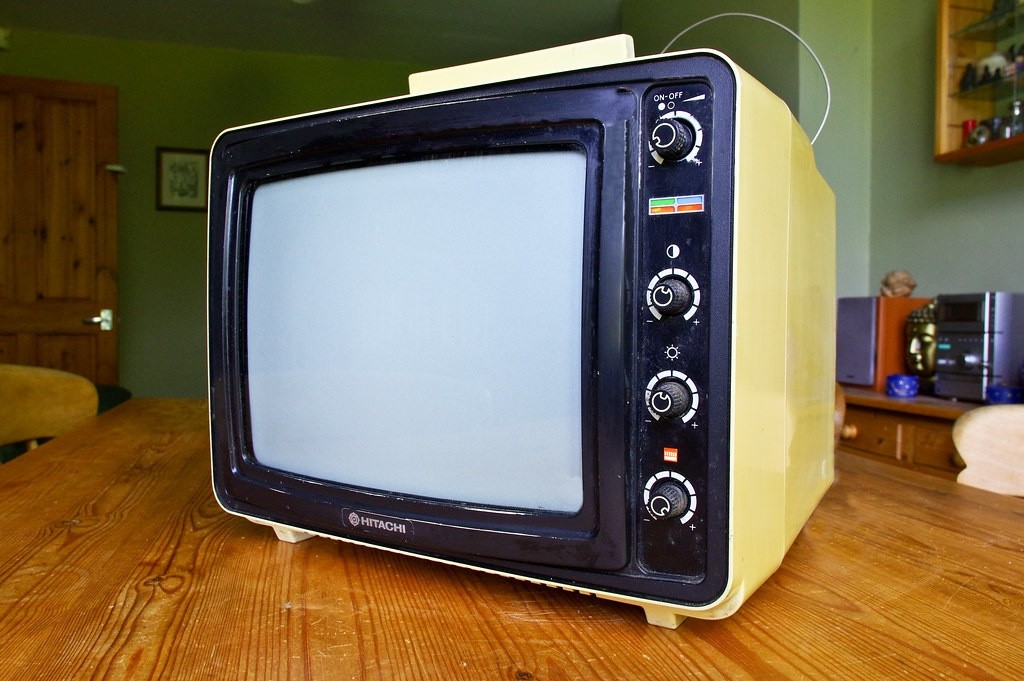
[933,0,1024,167]
[837,403,966,482]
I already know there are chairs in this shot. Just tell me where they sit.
[0,361,99,451]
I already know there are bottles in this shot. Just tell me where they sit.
[1000,100,1024,138]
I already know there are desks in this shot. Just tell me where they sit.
[0,395,1023,680]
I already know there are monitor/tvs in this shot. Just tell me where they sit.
[205,47,832,631]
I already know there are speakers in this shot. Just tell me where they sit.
[836,297,934,393]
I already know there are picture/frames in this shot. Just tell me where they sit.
[155,146,210,211]
